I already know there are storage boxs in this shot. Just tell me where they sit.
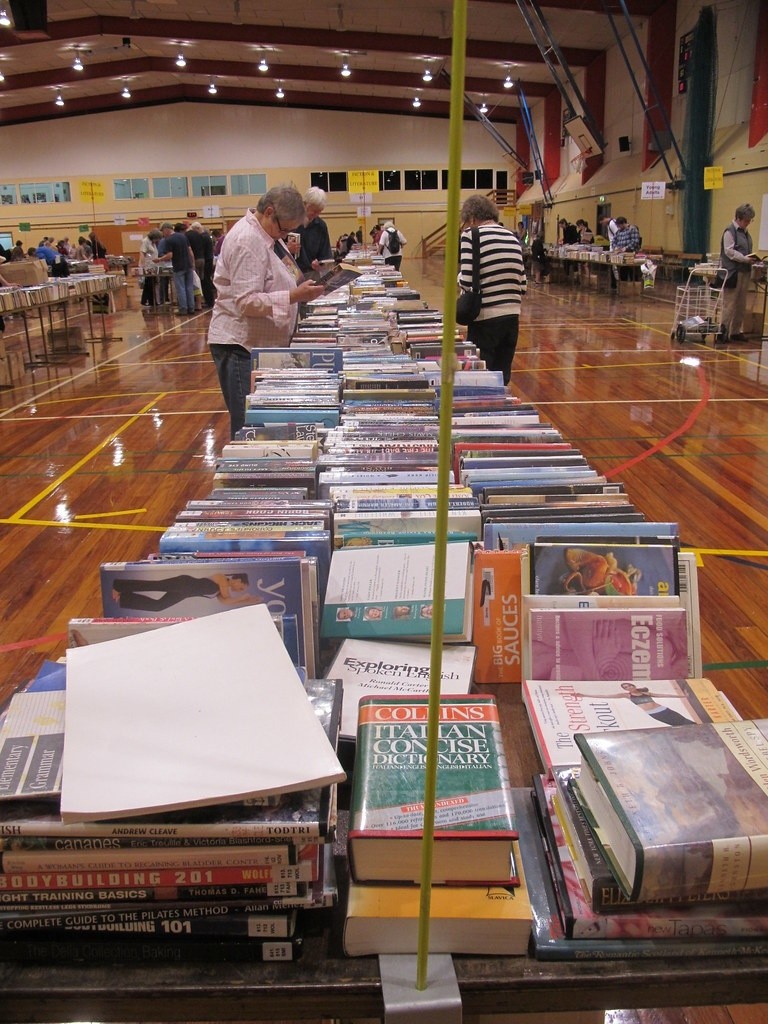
[0,259,49,287]
[114,288,129,312]
[616,280,644,298]
[47,326,85,351]
[739,312,763,334]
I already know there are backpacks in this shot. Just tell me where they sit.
[385,228,402,253]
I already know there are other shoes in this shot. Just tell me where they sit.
[716,334,731,343]
[175,307,195,315]
[729,333,748,342]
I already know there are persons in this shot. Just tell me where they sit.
[0,232,107,285]
[364,608,383,620]
[206,186,326,443]
[393,605,415,620]
[514,221,527,244]
[531,230,547,286]
[278,187,335,320]
[612,216,640,281]
[420,605,433,620]
[595,215,619,290]
[137,221,228,316]
[369,221,407,271]
[456,193,528,386]
[570,682,697,727]
[334,226,363,261]
[558,218,578,277]
[715,203,759,345]
[573,219,595,274]
[111,571,264,612]
[337,607,356,621]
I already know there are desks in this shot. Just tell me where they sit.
[690,272,768,341]
[522,254,645,297]
[0,285,136,392]
[131,273,178,316]
[0,350,25,386]
[1,682,768,1024]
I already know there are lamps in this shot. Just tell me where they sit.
[55,49,514,113]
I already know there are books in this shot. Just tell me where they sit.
[284,231,301,260]
[246,240,548,425]
[0,257,768,962]
[690,252,767,280]
[549,242,666,267]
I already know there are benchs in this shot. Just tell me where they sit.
[640,245,703,283]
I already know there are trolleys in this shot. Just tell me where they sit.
[670,267,729,344]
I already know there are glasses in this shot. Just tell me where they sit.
[270,201,299,234]
[461,219,467,231]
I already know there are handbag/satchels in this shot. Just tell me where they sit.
[455,290,481,326]
[713,269,738,288]
